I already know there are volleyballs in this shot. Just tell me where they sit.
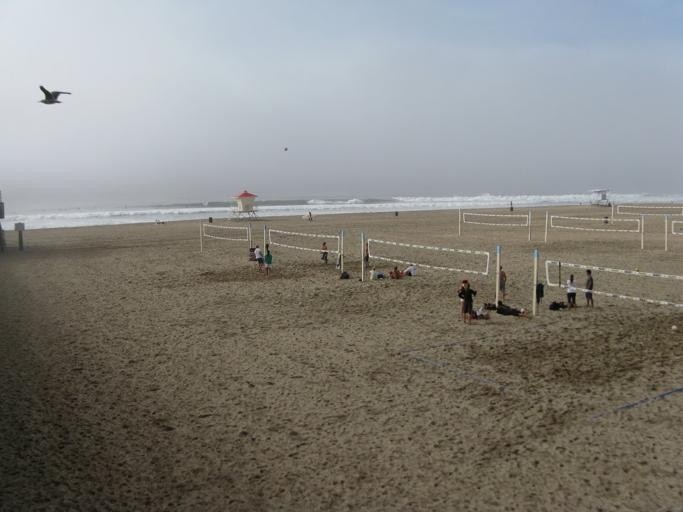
[285,148,287,150]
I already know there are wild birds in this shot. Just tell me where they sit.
[39,86,71,104]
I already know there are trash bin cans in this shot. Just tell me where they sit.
[603,216,608,224]
[209,217,212,223]
[249,248,257,261]
[395,212,398,216]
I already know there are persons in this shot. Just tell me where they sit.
[368,267,379,280]
[252,244,264,273]
[402,263,415,277]
[458,279,477,325]
[389,265,405,279]
[319,240,328,262]
[456,285,467,322]
[584,269,594,308]
[362,242,370,268]
[498,264,507,303]
[263,249,273,278]
[565,272,577,313]
